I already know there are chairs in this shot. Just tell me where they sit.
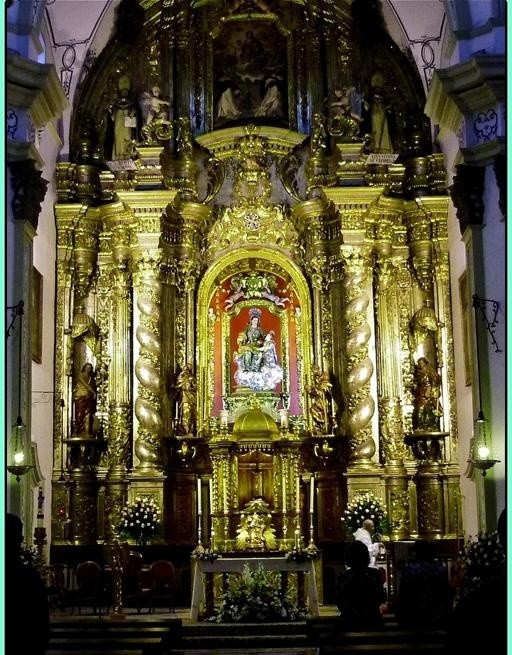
[69,559,175,621]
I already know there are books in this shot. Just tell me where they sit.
[123,116,138,127]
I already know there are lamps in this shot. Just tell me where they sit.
[8,300,35,481]
[467,292,501,478]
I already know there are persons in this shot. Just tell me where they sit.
[72,363,97,438]
[104,88,140,162]
[7,514,52,655]
[330,87,367,122]
[238,313,264,373]
[336,540,385,633]
[412,357,442,430]
[441,508,506,655]
[143,85,172,125]
[213,30,288,121]
[255,331,278,368]
[389,539,455,637]
[303,373,339,431]
[353,518,384,568]
[177,364,197,434]
